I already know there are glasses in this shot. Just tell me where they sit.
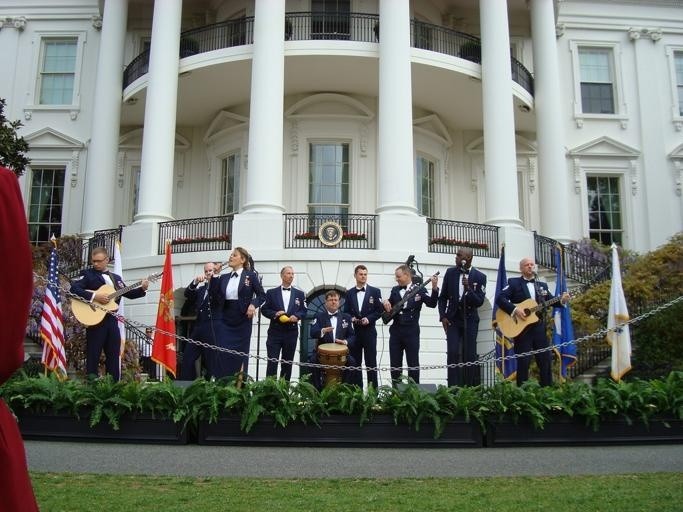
[327,298,340,302]
[91,255,108,263]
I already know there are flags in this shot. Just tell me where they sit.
[490,255,520,380]
[546,248,581,381]
[606,247,637,378]
[148,243,181,379]
[37,245,69,376]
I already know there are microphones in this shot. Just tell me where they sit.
[461,259,466,268]
[532,264,539,277]
[212,259,228,272]
[259,275,263,289]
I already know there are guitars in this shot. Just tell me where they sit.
[495,280,580,338]
[70,270,166,329]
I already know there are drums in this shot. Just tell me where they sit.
[316,342,350,386]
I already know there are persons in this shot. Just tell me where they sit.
[438,247,488,389]
[496,258,570,386]
[69,248,150,381]
[213,247,268,385]
[380,266,441,387]
[258,265,307,386]
[308,290,356,385]
[183,263,216,377]
[2,168,45,512]
[342,265,383,387]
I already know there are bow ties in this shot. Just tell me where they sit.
[327,312,338,318]
[399,285,407,290]
[355,287,365,293]
[459,269,470,274]
[525,279,535,283]
[283,287,291,291]
